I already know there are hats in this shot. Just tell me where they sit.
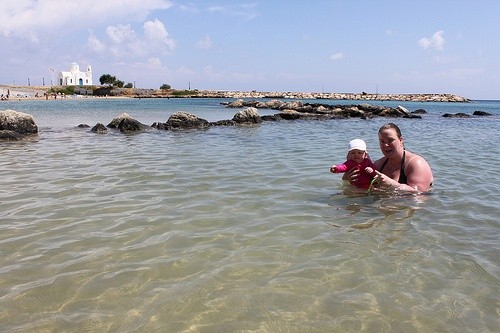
[347,139,367,152]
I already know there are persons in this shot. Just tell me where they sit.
[330,139,376,191]
[0,82,171,100]
[369,123,433,197]
